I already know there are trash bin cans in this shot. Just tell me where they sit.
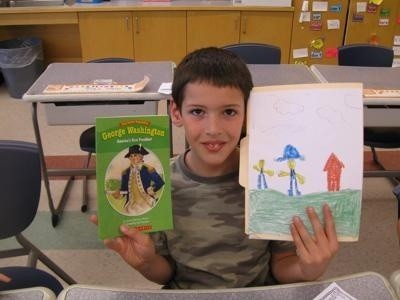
[0,38,44,98]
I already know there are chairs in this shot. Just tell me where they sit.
[220,42,282,66]
[1,139,78,300]
[77,58,134,213]
[337,43,400,171]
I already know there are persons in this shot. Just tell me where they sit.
[113,145,164,215]
[89,46,339,289]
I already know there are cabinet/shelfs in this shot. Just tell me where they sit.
[288,0,400,65]
[186,5,295,64]
[79,4,187,62]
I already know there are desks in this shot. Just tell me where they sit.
[308,62,400,178]
[56,267,400,300]
[20,60,176,229]
[249,62,323,94]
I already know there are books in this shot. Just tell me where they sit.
[94,114,173,240]
